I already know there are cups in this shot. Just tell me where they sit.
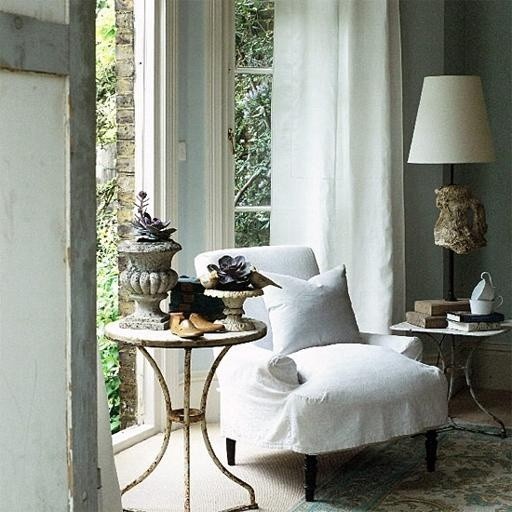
[471,272,498,303]
[469,296,504,316]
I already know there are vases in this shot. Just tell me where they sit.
[114,245,183,332]
[204,286,264,331]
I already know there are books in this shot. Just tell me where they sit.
[446,320,501,332]
[446,310,504,323]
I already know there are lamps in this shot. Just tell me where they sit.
[407,74,498,331]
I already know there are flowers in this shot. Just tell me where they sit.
[122,189,179,243]
[208,251,255,287]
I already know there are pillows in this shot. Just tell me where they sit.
[259,264,364,356]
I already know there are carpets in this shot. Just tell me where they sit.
[282,415,512,512]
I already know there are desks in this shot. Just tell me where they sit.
[390,314,511,435]
[104,312,267,511]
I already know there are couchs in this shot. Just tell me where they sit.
[193,246,463,500]
